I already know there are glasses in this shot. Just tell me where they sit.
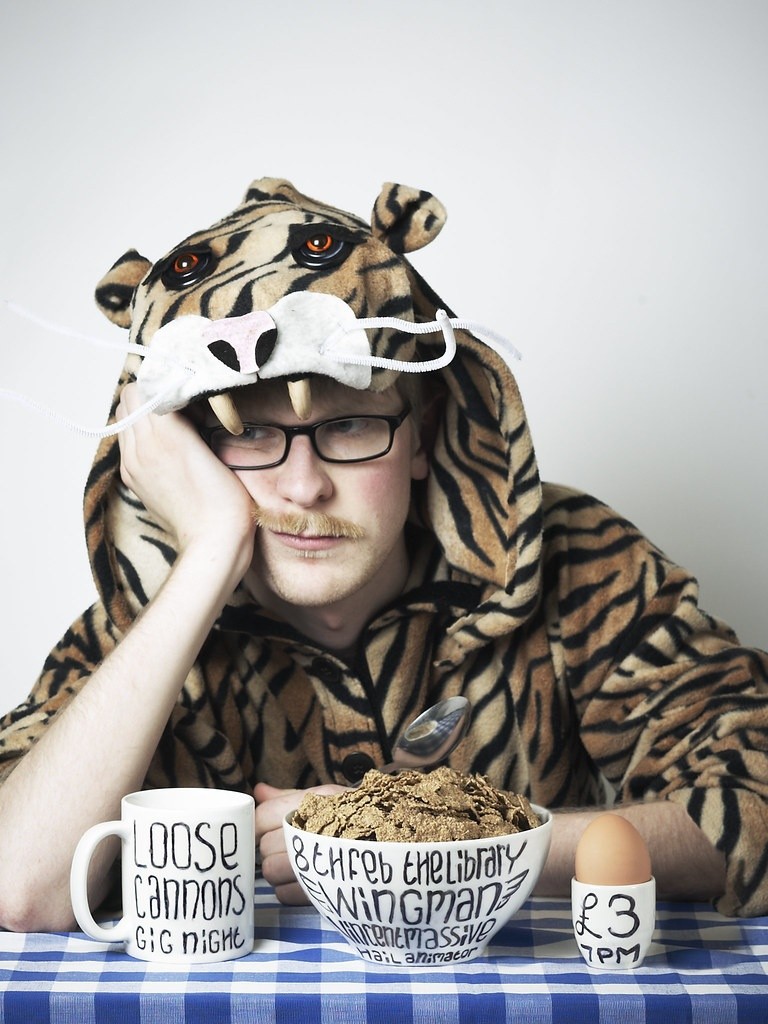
[196,402,412,470]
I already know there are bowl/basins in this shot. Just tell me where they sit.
[283,803,553,966]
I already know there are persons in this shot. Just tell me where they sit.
[0,175,767,932]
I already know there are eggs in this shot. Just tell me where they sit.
[574,814,651,885]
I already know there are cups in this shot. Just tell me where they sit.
[572,874,656,973]
[69,788,257,963]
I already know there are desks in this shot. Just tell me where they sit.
[0,863,768,1024]
